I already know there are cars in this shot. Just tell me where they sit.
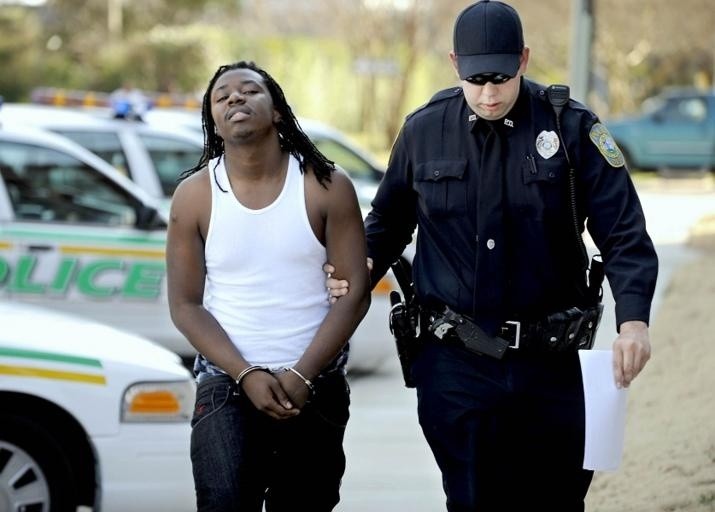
[605,93,715,171]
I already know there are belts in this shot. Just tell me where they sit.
[430,311,570,349]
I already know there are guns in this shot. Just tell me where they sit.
[389,293,422,390]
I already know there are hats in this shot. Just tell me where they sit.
[453,0,524,80]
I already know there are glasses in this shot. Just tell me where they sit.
[467,73,512,84]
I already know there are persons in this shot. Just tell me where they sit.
[167,61,371,512]
[324,0,658,512]
[107,78,150,126]
